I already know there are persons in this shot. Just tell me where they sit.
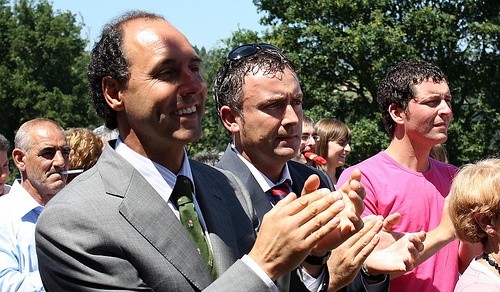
[0,119,69,292]
[0,133,9,196]
[335,61,482,292]
[65,125,119,171]
[450,158,500,292]
[292,113,352,185]
[36,12,365,292]
[215,45,427,292]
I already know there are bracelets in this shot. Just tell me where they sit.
[360,267,385,282]
[304,250,331,265]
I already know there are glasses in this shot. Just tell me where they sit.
[300,135,320,145]
[218,43,279,90]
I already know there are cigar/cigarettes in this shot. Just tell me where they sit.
[61,170,83,174]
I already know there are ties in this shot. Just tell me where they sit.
[270,179,290,205]
[170,175,220,283]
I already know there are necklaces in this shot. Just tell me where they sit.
[482,252,500,274]
